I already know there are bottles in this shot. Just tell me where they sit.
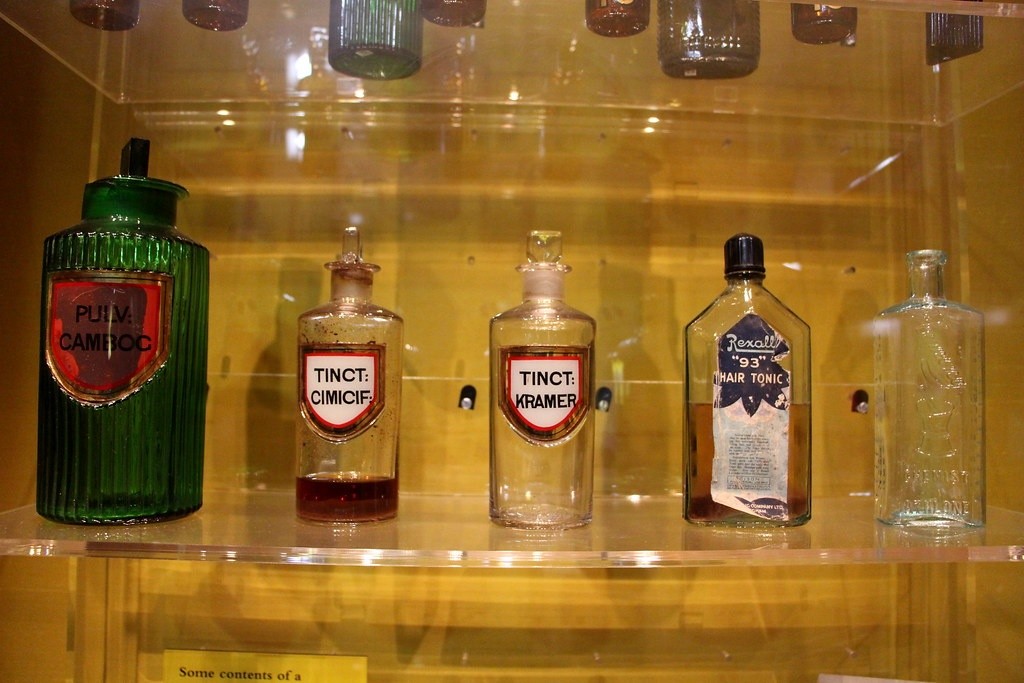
[585,0,651,37]
[874,248,987,536]
[69,0,140,31]
[657,0,760,79]
[791,3,857,44]
[423,0,487,27]
[489,231,595,531]
[36,135,209,526]
[182,0,248,32]
[296,225,403,523]
[925,1,983,65]
[328,0,423,82]
[685,233,812,532]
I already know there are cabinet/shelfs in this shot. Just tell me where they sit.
[0,0,1024,683]
